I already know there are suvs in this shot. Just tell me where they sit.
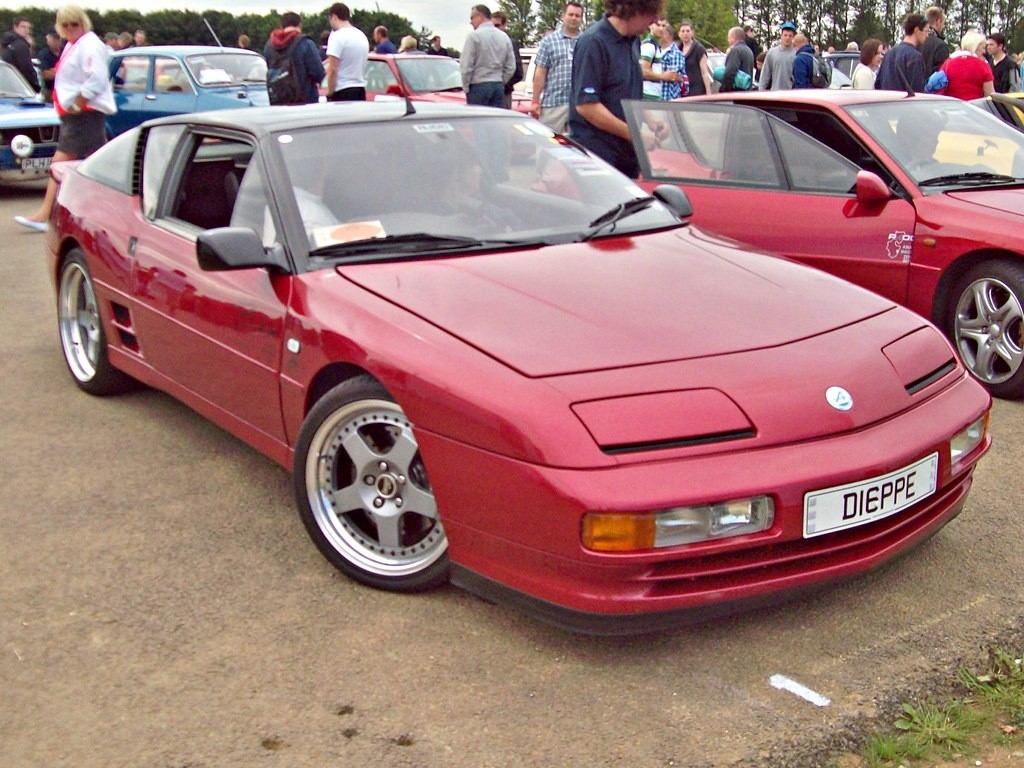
[825,51,886,81]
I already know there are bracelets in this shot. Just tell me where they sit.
[532,100,539,104]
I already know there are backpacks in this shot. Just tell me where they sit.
[799,51,832,87]
[265,34,313,106]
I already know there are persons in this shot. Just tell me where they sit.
[637,13,714,100]
[94,229,197,316]
[113,62,126,90]
[525,2,584,133]
[459,4,523,109]
[103,28,150,50]
[986,32,1024,94]
[237,33,250,49]
[568,0,670,179]
[719,22,834,90]
[10,5,116,232]
[259,138,340,249]
[874,7,949,93]
[846,39,896,89]
[373,27,448,55]
[935,31,995,101]
[897,108,941,174]
[263,2,369,102]
[0,15,63,103]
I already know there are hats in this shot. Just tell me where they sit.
[104,32,118,39]
[782,23,796,30]
[398,35,418,53]
[847,41,858,51]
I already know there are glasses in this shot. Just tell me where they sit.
[61,22,80,28]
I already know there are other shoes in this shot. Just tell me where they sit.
[14,215,47,233]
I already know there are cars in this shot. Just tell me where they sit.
[314,55,541,157]
[705,53,760,88]
[0,60,64,182]
[540,90,1024,401]
[102,46,271,140]
[444,48,538,96]
[45,101,993,634]
[890,93,1024,179]
